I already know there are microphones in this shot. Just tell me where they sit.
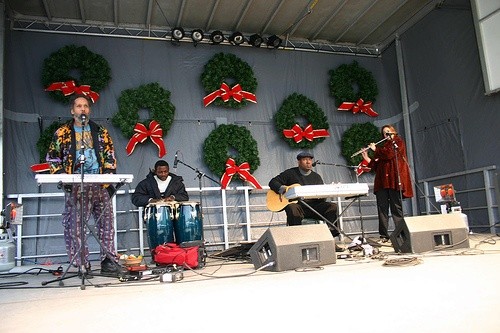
[311,160,318,166]
[173,152,178,169]
[80,113,86,125]
[386,133,398,136]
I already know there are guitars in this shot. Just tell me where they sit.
[265,183,301,212]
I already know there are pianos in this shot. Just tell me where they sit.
[35,171,136,283]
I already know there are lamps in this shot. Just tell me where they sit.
[191,29,204,47]
[267,35,282,48]
[209,30,224,44]
[171,26,185,46]
[228,31,244,46]
[248,34,263,48]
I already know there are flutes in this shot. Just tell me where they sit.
[350,136,389,159]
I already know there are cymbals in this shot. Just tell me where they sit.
[284,182,370,254]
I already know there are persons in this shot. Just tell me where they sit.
[361,125,414,243]
[268,152,339,237]
[131,160,189,207]
[46,94,121,278]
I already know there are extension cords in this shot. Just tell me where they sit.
[160,272,184,282]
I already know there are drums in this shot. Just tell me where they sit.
[146,202,174,255]
[174,200,202,244]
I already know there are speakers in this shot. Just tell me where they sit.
[389,213,470,254]
[249,223,337,270]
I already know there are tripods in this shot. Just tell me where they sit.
[197,173,229,267]
[41,124,133,290]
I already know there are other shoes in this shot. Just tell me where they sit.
[335,243,345,252]
[377,237,388,244]
[100,257,128,276]
[78,263,92,279]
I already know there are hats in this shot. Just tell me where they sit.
[297,152,314,161]
